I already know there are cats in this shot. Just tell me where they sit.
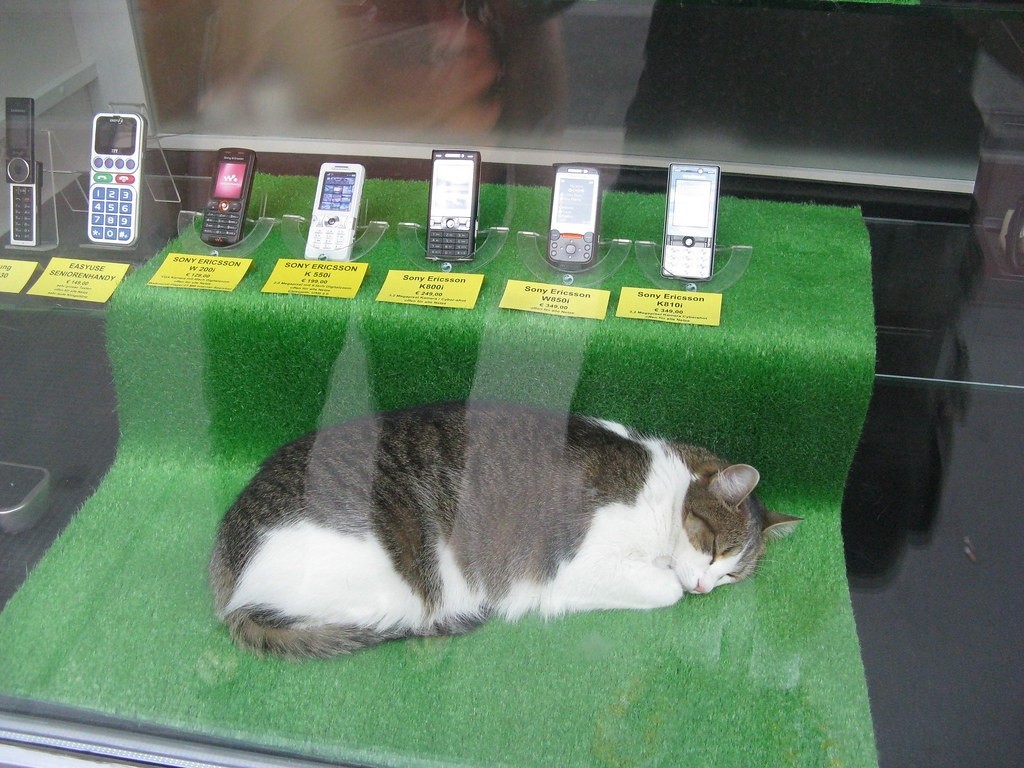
[204,398,804,668]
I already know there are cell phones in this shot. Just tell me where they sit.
[547,166,602,269]
[425,149,482,262]
[201,147,256,246]
[87,112,147,247]
[305,163,366,261]
[660,163,721,282]
[5,97,44,248]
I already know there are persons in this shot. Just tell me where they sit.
[139,1,1024,677]
